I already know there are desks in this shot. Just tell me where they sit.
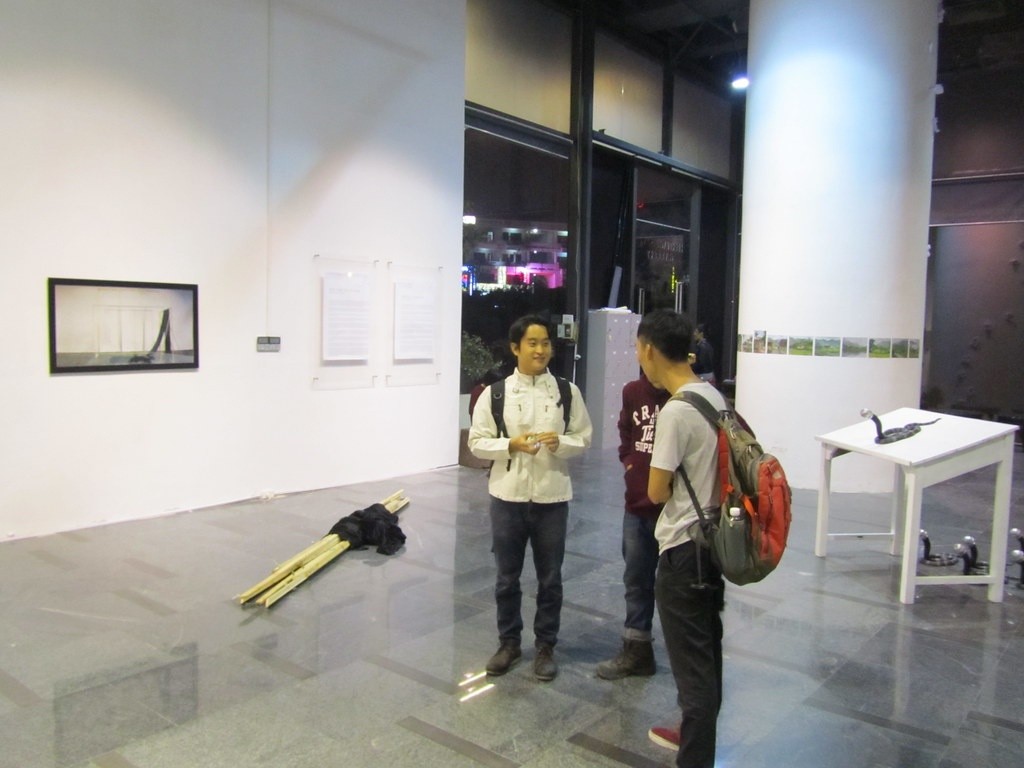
[814,404,1021,607]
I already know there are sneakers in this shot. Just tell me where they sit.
[535,639,558,680]
[647,721,680,753]
[486,638,521,675]
[597,638,656,681]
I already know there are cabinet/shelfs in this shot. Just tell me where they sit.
[584,309,643,451]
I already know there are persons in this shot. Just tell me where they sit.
[596,373,673,679]
[467,315,593,681]
[637,308,726,767]
[695,323,713,373]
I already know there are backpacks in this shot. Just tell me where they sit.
[667,390,792,589]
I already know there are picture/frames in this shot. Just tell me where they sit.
[46,275,201,377]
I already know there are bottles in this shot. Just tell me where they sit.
[726,506,745,525]
